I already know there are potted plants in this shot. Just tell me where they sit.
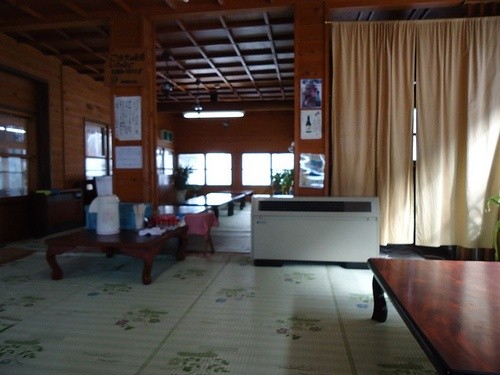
[174,166,197,203]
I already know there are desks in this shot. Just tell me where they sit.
[45,226,189,285]
[184,190,254,218]
[158,205,210,214]
[368,258,500,375]
